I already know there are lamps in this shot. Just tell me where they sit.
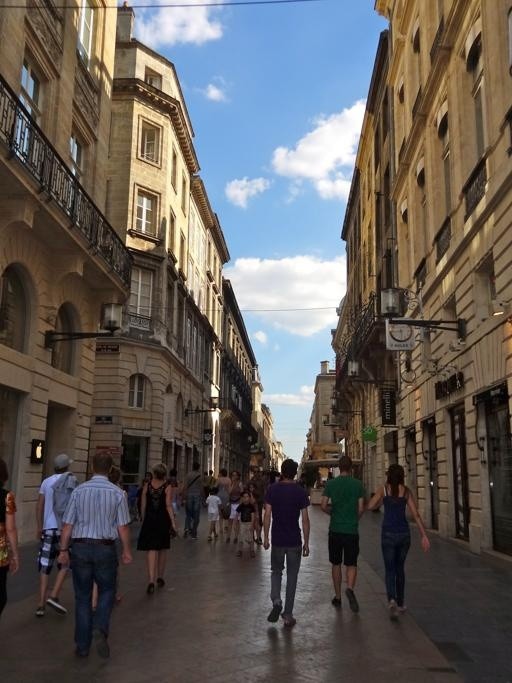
[320,414,349,430]
[329,395,362,415]
[347,357,399,390]
[184,396,221,419]
[380,286,467,343]
[44,299,121,349]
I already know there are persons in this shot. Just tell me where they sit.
[318,455,367,617]
[56,449,134,658]
[33,452,83,617]
[1,456,21,615]
[91,464,280,622]
[366,464,431,621]
[263,456,312,627]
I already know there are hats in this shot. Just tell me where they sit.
[53,453,75,467]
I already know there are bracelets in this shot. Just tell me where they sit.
[59,548,70,553]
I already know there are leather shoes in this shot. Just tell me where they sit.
[91,627,111,658]
[73,643,90,656]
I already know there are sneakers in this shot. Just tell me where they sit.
[281,611,296,624]
[46,596,68,617]
[398,606,408,614]
[146,583,155,593]
[388,600,399,621]
[345,587,357,601]
[33,605,45,616]
[182,526,263,558]
[157,577,166,586]
[267,603,283,622]
[331,596,342,606]
